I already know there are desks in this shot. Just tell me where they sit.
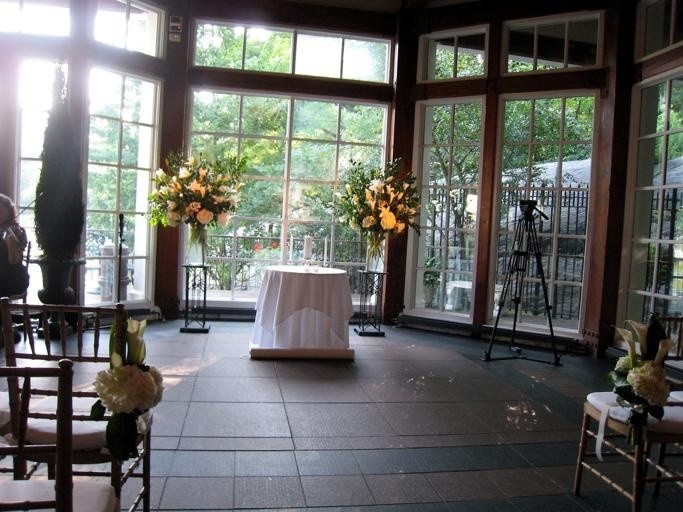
[354,269,387,336]
[249,264,354,353]
[180,264,211,333]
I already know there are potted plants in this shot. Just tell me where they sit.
[422,257,442,306]
[26,61,88,337]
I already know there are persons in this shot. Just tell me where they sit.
[0,194,30,347]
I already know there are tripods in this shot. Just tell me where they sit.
[483,216,561,366]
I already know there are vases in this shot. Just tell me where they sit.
[365,231,384,272]
[185,224,209,265]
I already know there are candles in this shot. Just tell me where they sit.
[304,236,312,259]
[324,237,327,264]
[289,235,293,262]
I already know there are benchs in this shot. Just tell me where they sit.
[445,281,504,316]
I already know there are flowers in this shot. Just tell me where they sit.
[319,157,421,260]
[147,152,249,244]
[606,314,675,424]
[88,315,164,460]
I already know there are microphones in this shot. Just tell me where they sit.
[119,214,124,236]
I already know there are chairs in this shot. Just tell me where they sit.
[0,361,118,512]
[572,312,683,512]
[0,298,150,512]
